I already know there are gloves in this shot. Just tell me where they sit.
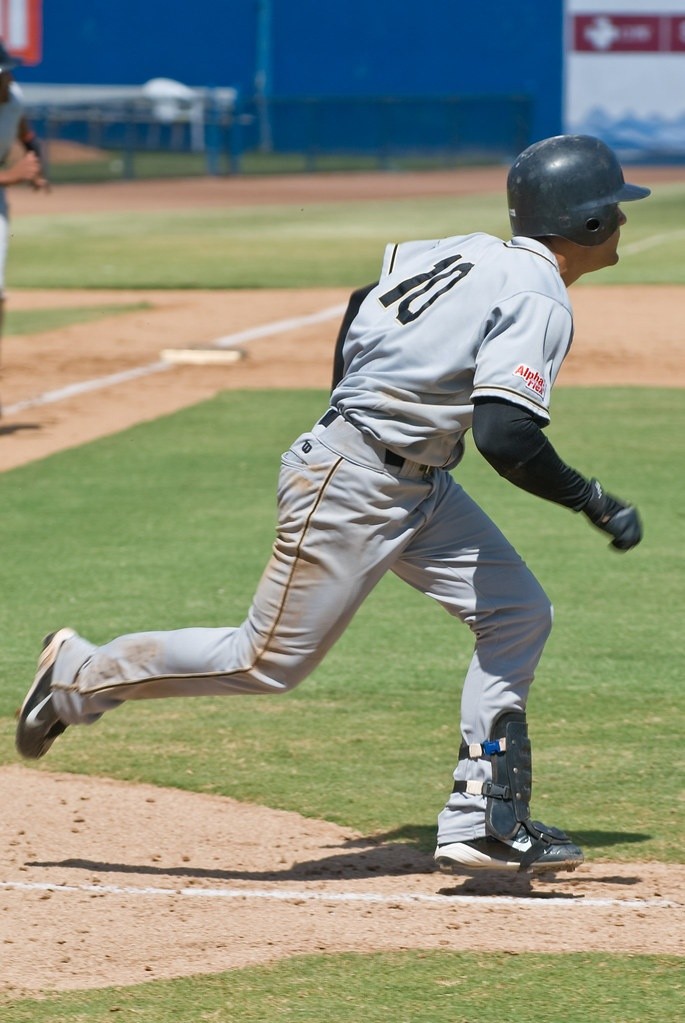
[582,476,644,551]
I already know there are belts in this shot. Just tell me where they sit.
[319,410,435,475]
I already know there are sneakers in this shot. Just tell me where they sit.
[434,835,584,874]
[14,625,80,759]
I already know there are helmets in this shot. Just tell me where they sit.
[0,41,23,76]
[506,134,651,247]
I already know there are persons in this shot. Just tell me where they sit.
[15,133,652,879]
[0,40,51,320]
